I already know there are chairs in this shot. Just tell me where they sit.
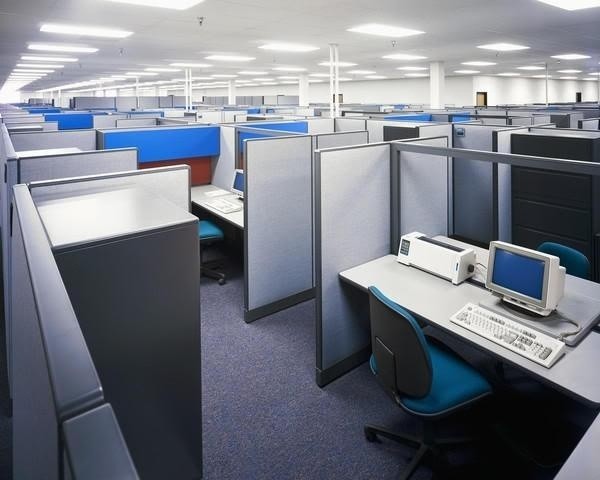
[536,242,590,278]
[189,203,228,286]
[362,285,496,480]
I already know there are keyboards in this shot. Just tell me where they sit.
[205,197,241,215]
[448,302,568,369]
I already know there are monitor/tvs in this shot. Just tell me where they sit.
[483,239,568,320]
[228,169,247,200]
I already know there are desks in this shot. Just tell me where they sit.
[188,184,244,230]
[337,234,599,409]
